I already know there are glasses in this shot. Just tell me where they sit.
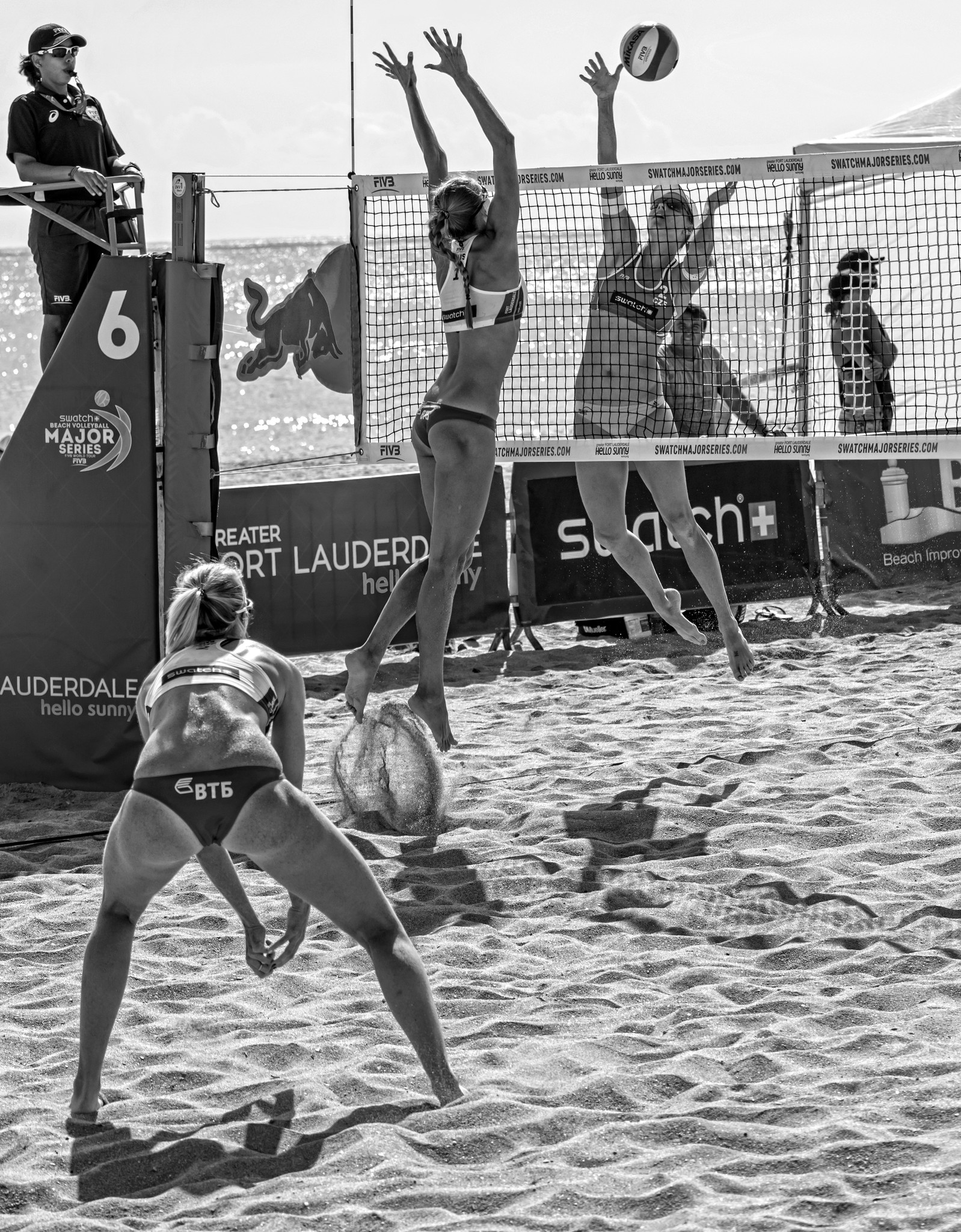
[467,185,489,224]
[232,598,254,615]
[29,45,79,59]
[650,197,691,223]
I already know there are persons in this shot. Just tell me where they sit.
[345,26,527,753]
[573,52,756,682]
[6,23,145,373]
[825,249,899,435]
[70,557,463,1115]
[655,301,788,438]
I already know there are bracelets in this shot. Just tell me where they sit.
[69,165,81,181]
[122,161,141,175]
[682,263,706,282]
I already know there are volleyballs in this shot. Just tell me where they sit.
[620,21,679,82]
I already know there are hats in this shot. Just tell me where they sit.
[828,269,877,298]
[837,249,886,271]
[650,184,700,230]
[28,23,87,54]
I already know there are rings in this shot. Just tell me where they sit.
[140,172,143,174]
